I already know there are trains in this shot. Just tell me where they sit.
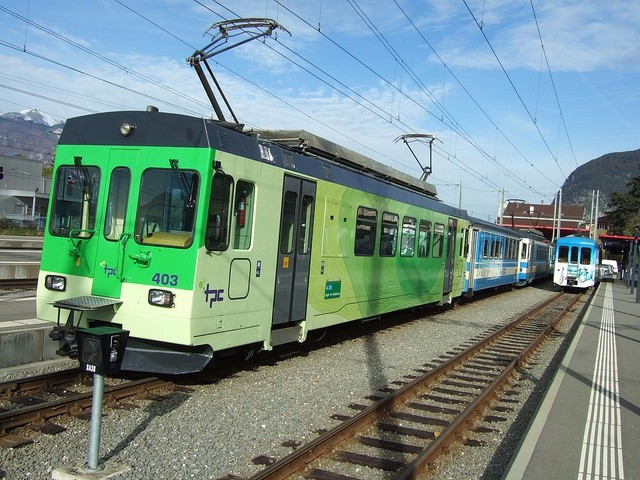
[553,237,602,288]
[36,104,556,374]
